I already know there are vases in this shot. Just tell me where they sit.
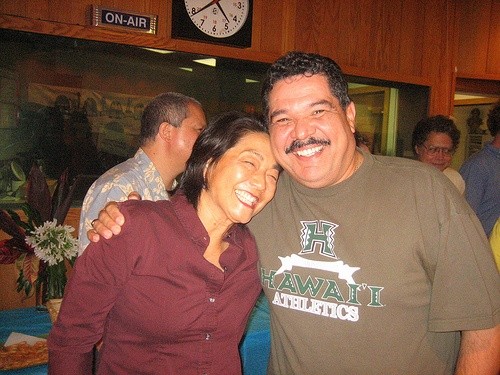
[36,260,56,313]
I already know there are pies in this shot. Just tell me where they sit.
[0,340,49,370]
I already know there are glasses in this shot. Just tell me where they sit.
[421,142,455,156]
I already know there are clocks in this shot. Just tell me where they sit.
[171,0,253,49]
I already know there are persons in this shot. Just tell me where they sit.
[460,101,500,240]
[466,108,483,133]
[413,114,467,196]
[46,109,283,375]
[78,93,208,257]
[352,130,372,154]
[489,216,500,275]
[86,51,500,375]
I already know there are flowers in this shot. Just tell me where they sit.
[0,160,78,303]
